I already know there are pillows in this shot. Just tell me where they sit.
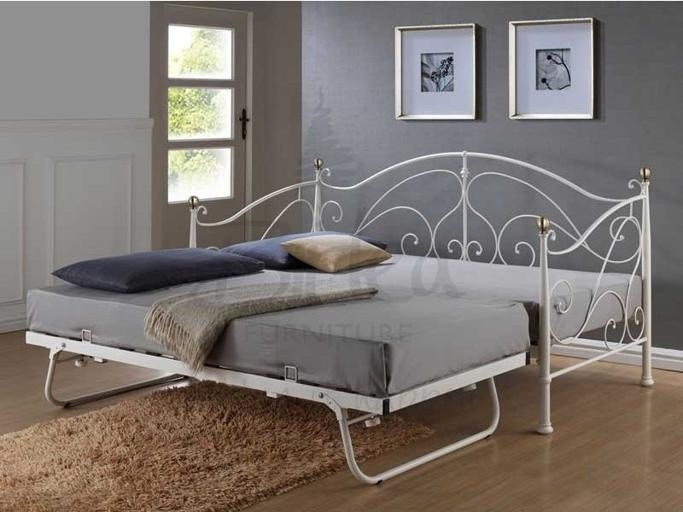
[47,229,396,297]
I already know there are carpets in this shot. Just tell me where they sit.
[1,365,431,511]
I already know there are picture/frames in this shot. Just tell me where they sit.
[508,16,597,119]
[395,22,478,121]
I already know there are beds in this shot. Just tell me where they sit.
[25,147,654,486]
